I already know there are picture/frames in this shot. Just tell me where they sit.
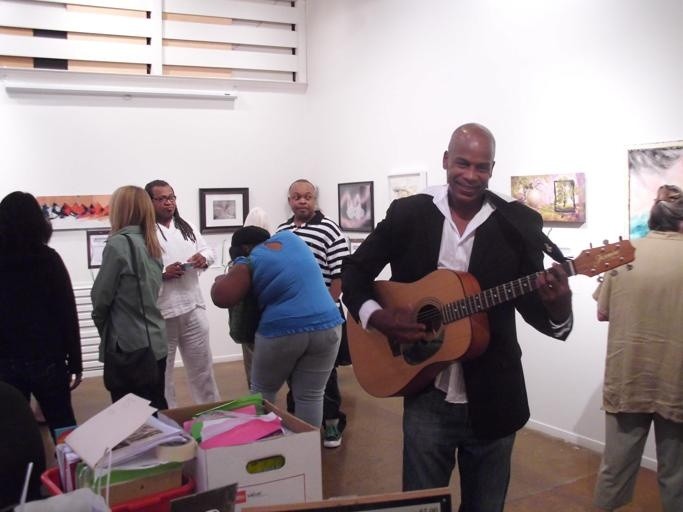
[384,168,428,209]
[83,229,111,270]
[197,186,250,235]
[336,180,376,235]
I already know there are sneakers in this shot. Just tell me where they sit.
[323,416,349,448]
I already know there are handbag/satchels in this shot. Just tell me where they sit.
[103,346,161,392]
[227,256,261,344]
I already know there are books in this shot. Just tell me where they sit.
[50,394,196,505]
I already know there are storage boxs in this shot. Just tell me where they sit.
[39,465,196,512]
[156,393,324,511]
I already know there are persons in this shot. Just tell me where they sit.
[0,383,48,509]
[341,121,573,512]
[146,179,218,408]
[593,183,682,509]
[1,189,82,434]
[275,176,349,447]
[209,224,341,428]
[87,187,166,409]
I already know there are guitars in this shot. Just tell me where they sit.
[345,235,636,399]
